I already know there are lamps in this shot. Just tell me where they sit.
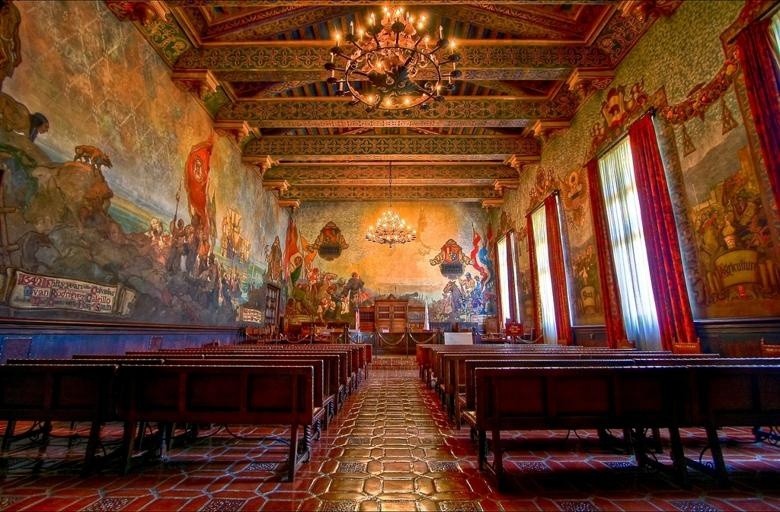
[324,0,461,117]
[365,160,417,247]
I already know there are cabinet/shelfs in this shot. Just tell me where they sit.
[374,299,408,358]
